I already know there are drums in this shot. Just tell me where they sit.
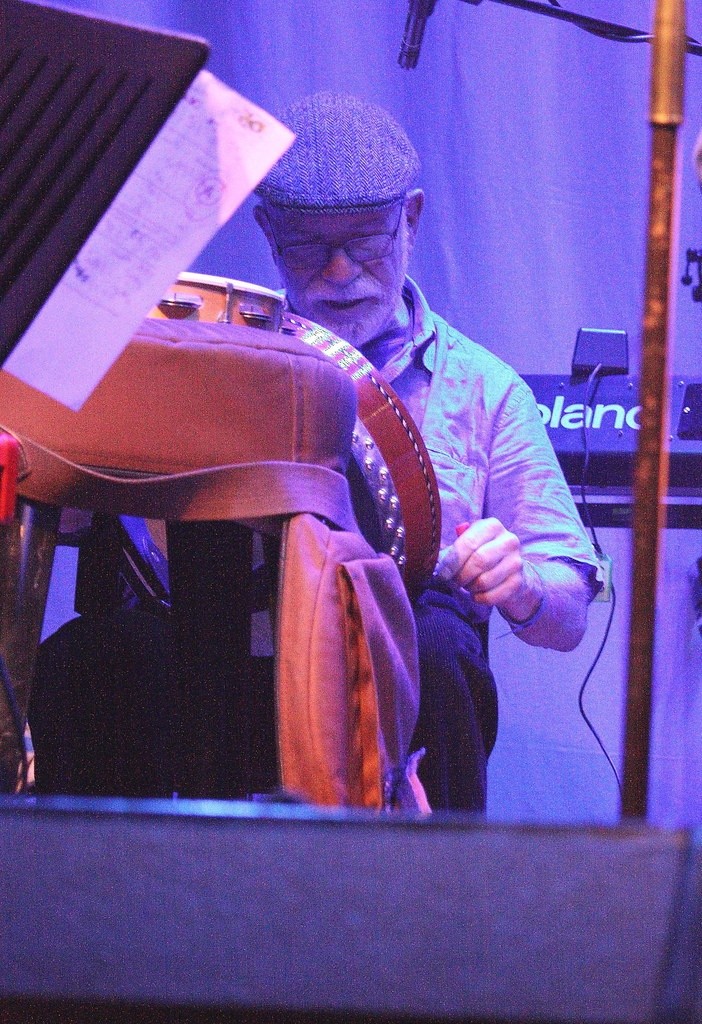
[143,269,286,336]
[279,314,441,607]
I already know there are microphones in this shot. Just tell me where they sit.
[397,0,433,69]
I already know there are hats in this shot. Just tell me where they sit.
[254,91,421,214]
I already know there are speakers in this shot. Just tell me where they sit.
[485,483,702,857]
[0,794,701,1023]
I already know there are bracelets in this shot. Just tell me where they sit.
[494,558,549,639]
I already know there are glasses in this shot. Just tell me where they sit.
[265,196,403,270]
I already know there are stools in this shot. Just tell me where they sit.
[1,320,359,797]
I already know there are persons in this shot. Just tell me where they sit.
[28,89,606,817]
